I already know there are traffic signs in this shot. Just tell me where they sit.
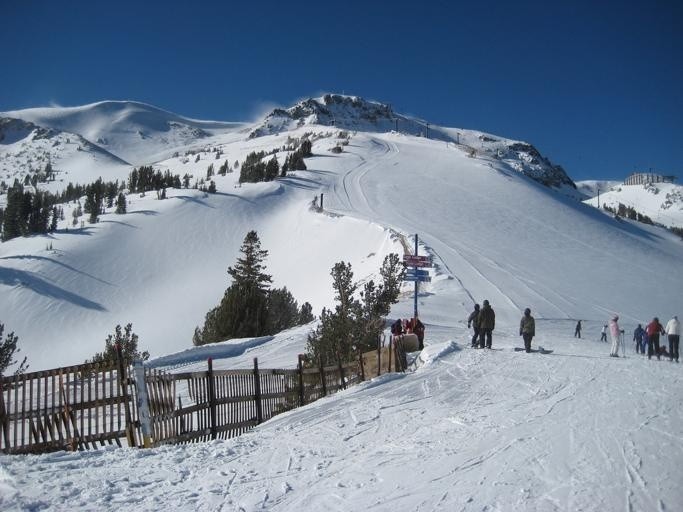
[402,254,432,282]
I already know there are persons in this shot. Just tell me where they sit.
[646,344,667,356]
[640,327,650,353]
[476,298,495,350]
[665,314,681,363]
[600,323,608,342]
[466,303,482,348]
[607,314,623,357]
[518,307,536,352]
[632,324,644,352]
[574,319,582,338]
[388,316,425,350]
[644,317,664,360]
